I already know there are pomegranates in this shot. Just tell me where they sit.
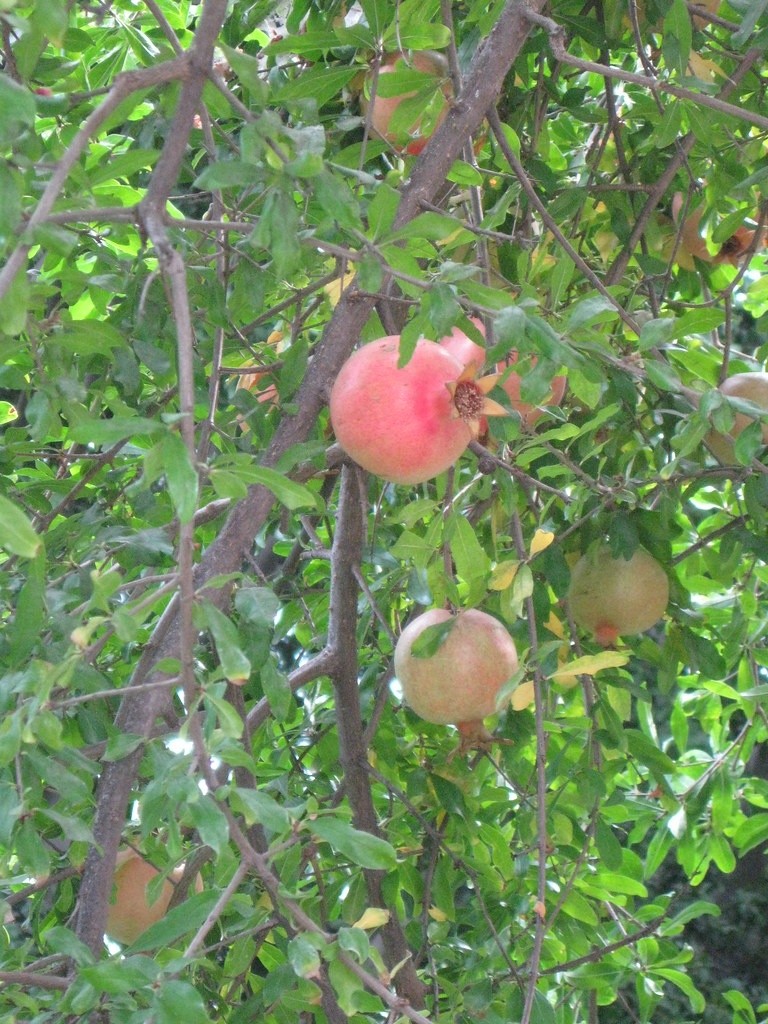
[103,840,205,946]
[232,1,768,744]
